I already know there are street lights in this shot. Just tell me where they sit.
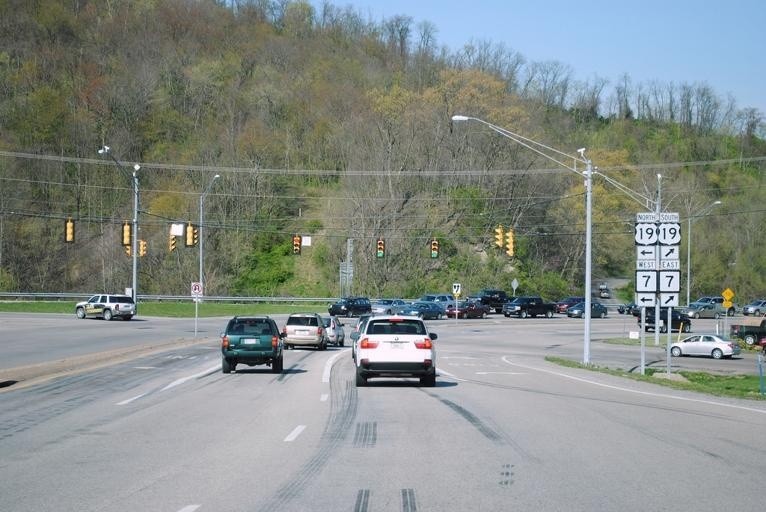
[449,112,593,366]
[686,200,723,307]
[579,169,663,348]
[199,175,220,304]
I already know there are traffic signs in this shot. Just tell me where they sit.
[660,213,678,222]
[638,247,655,270]
[637,214,658,222]
[660,246,679,269]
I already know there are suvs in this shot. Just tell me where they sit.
[75,293,136,321]
[220,314,284,373]
[330,297,373,318]
[349,314,438,387]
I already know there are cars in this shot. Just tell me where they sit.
[319,315,345,347]
[354,319,368,367]
[351,315,376,358]
[671,334,741,359]
[406,302,446,321]
[420,289,491,319]
[637,309,691,333]
[688,297,736,318]
[566,301,609,319]
[743,299,765,316]
[370,298,412,314]
[599,284,611,299]
[283,312,328,349]
[616,303,638,316]
[557,296,590,313]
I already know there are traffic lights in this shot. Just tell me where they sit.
[186,227,198,246]
[493,224,503,249]
[139,241,147,257]
[376,240,385,258]
[505,228,514,257]
[431,240,439,259]
[169,234,176,252]
[292,235,301,254]
[125,245,131,257]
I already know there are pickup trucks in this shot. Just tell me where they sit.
[503,295,557,318]
[466,289,516,314]
[731,319,766,346]
